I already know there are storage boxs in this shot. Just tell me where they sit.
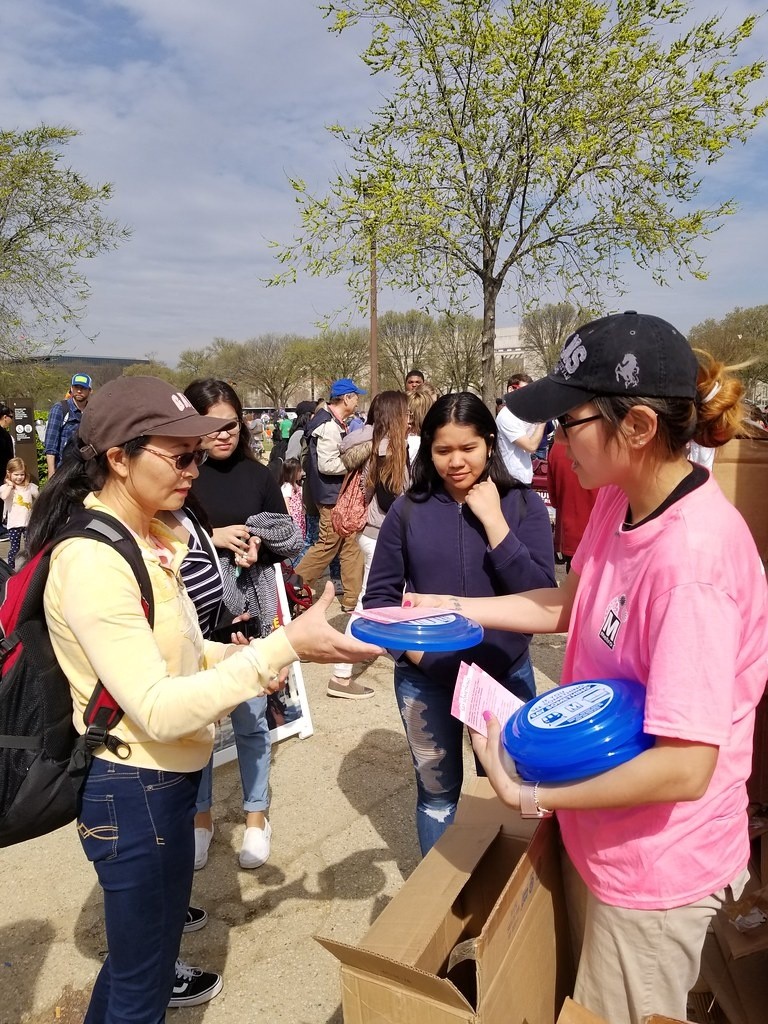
[310,777,768,1024]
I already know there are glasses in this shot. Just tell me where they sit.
[7,414,14,419]
[404,411,413,421]
[558,411,604,437]
[136,444,205,470]
[207,420,243,439]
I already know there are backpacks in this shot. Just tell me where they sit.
[0,509,154,849]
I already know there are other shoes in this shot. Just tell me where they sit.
[0,534,10,541]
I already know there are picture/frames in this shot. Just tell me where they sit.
[212,563,315,768]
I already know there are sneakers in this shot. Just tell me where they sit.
[167,959,223,1007]
[327,678,375,700]
[332,579,343,595]
[239,817,272,870]
[195,823,214,869]
[182,907,207,933]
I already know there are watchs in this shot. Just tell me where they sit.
[520,780,553,820]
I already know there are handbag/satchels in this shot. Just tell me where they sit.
[252,424,263,434]
[330,467,368,538]
[278,555,313,621]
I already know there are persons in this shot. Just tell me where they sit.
[272,371,601,699]
[363,394,555,857]
[28,376,384,1024]
[400,309,768,1023]
[0,375,288,1008]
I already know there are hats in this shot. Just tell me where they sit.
[72,372,91,388]
[505,311,699,423]
[79,376,236,460]
[330,379,366,400]
[295,401,318,414]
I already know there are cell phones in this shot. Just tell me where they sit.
[210,616,262,645]
